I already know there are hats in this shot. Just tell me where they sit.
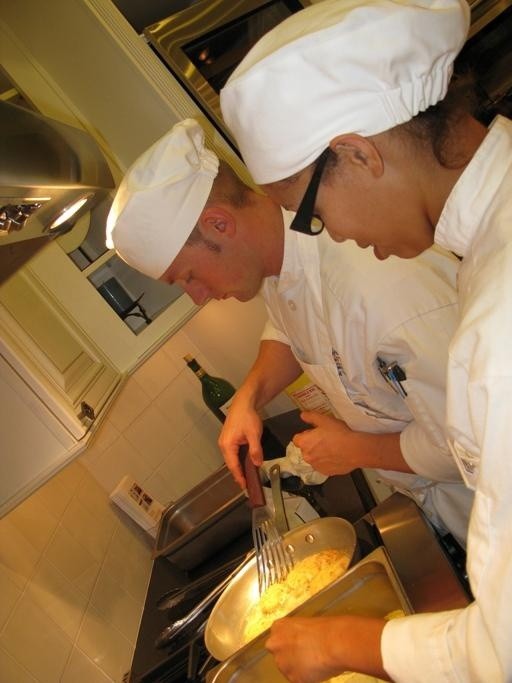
[105,117,220,282]
[219,1,472,186]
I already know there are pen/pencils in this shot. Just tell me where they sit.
[376,356,407,399]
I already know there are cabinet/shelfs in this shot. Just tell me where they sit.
[1,0,271,521]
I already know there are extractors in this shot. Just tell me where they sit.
[1,100,117,290]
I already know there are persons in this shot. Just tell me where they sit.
[213,1,512,681]
[100,110,477,567]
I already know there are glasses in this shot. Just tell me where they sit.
[289,148,333,236]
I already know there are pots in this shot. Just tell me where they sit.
[200,464,360,666]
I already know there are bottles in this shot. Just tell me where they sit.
[182,352,268,449]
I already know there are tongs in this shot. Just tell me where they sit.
[151,544,256,650]
